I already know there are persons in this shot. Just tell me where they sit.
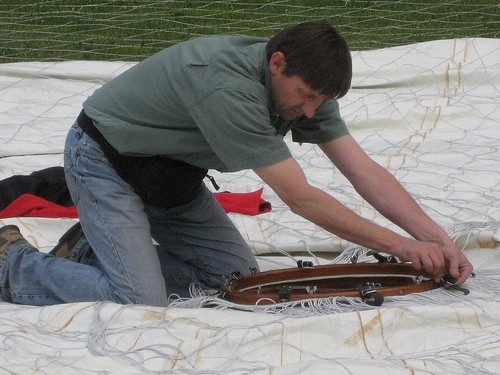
[0,20,473,308]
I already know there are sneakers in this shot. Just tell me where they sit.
[49,221,85,260]
[0,225,23,256]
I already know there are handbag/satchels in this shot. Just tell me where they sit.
[77,110,220,209]
